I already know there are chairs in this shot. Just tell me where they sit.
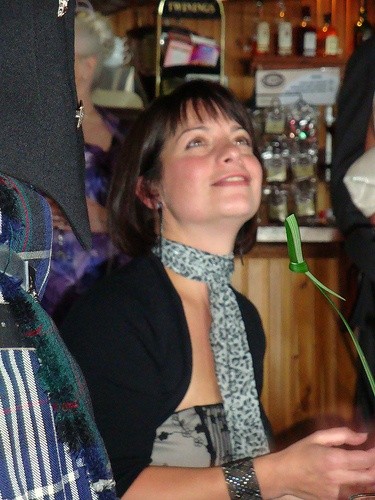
[0,173,120,500]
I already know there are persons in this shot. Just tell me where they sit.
[0,0,375,500]
[330,26,375,426]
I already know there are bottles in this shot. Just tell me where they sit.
[249,1,274,58]
[296,4,317,57]
[354,0,373,52]
[318,12,339,59]
[274,2,293,56]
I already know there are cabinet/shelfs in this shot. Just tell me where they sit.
[243,0,374,242]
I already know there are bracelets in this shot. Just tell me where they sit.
[221,458,264,500]
